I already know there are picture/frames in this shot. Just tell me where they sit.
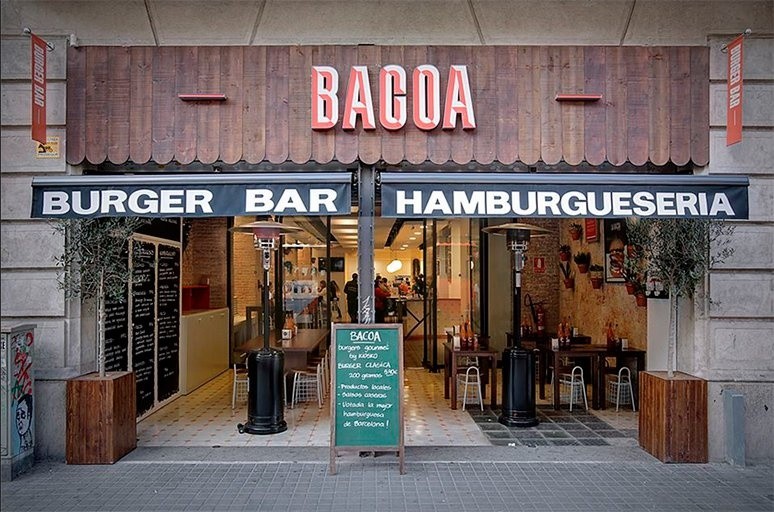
[604,216,630,284]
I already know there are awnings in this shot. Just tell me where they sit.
[30,173,351,219]
[380,172,749,219]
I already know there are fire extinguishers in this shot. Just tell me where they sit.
[524,294,548,338]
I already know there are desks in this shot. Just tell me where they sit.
[234,327,330,403]
[535,342,647,411]
[441,342,500,410]
[503,328,592,384]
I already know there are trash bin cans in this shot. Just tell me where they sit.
[0,322,38,483]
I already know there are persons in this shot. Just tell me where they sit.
[264,276,434,323]
[344,273,358,323]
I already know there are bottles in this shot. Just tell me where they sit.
[281,311,292,339]
[473,333,478,351]
[460,315,465,335]
[607,323,614,349]
[568,315,573,336]
[464,314,470,335]
[561,316,566,332]
[557,322,564,348]
[565,323,571,348]
[614,324,621,350]
[289,311,295,338]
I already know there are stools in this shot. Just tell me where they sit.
[548,364,589,412]
[605,366,636,412]
[231,345,331,409]
[457,356,485,412]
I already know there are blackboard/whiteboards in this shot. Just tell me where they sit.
[330,320,405,451]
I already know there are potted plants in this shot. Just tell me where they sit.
[557,244,571,261]
[559,261,575,289]
[589,265,604,289]
[47,216,158,466]
[611,215,737,464]
[573,251,591,273]
[619,267,647,308]
[568,222,583,241]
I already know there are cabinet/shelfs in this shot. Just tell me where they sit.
[179,307,229,395]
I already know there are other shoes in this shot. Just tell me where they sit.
[337,315,342,319]
